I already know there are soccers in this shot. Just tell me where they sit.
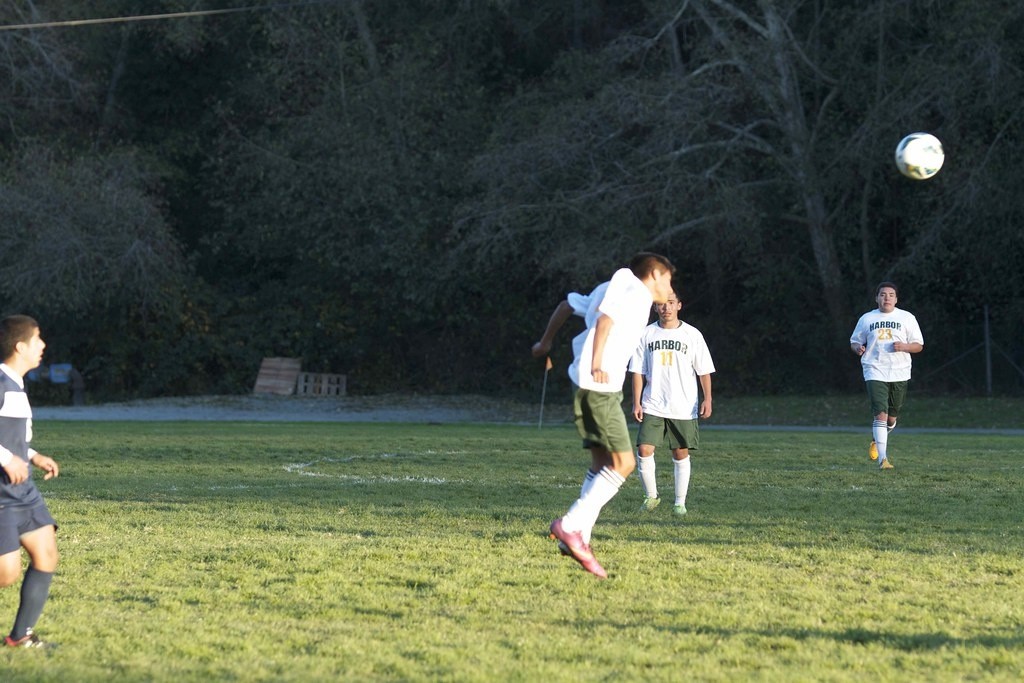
[894,132,946,180]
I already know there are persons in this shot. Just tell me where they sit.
[628,293,716,516]
[532,252,676,580]
[0,314,60,649]
[850,283,925,470]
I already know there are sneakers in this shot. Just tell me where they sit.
[550,516,608,578]
[671,505,688,519]
[3,628,61,659]
[638,492,663,515]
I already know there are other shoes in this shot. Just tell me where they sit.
[869,440,879,460]
[879,457,894,470]
